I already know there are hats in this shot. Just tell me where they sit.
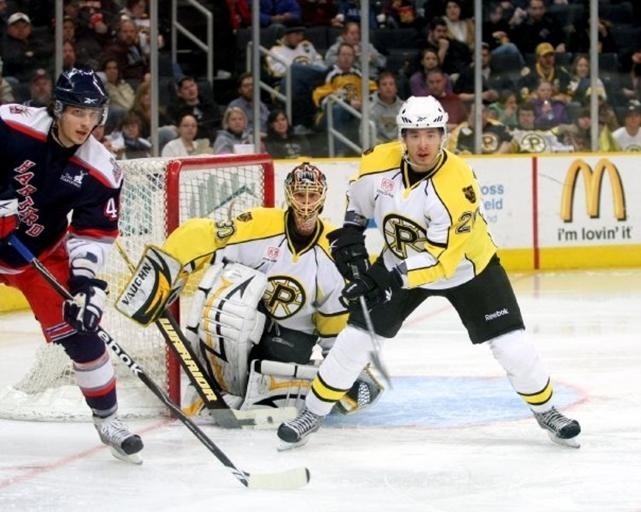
[281,17,307,34]
[7,12,31,26]
[625,99,641,113]
[536,41,556,57]
[31,67,48,83]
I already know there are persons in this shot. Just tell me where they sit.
[0,68,144,456]
[260,1,362,159]
[0,0,641,152]
[277,96,580,443]
[112,161,385,427]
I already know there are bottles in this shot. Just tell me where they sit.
[542,99,555,120]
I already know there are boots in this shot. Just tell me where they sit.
[91,410,144,465]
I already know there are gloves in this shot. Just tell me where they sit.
[338,267,405,311]
[325,226,371,282]
[61,277,109,335]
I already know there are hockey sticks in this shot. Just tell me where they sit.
[113,237,299,431]
[358,292,394,393]
[4,234,310,493]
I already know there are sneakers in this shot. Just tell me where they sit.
[274,406,323,453]
[529,404,582,449]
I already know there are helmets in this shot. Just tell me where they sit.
[53,67,110,127]
[396,94,450,169]
[284,162,327,233]
